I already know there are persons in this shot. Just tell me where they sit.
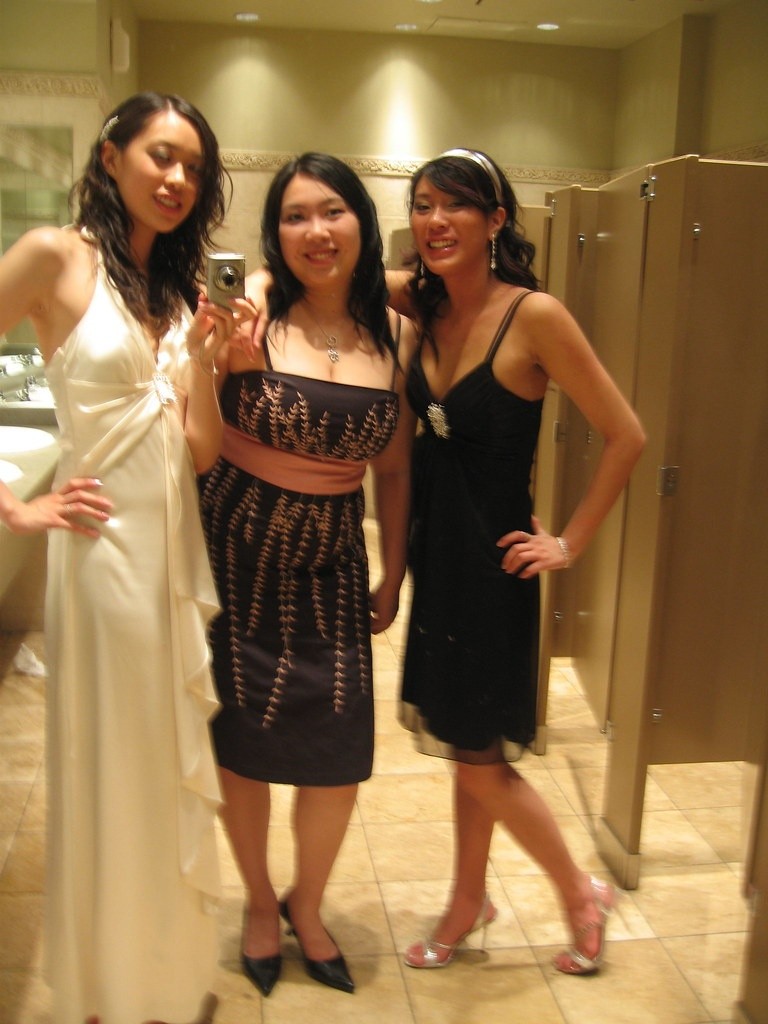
[385,149,647,976]
[195,152,416,997]
[0,94,259,1024]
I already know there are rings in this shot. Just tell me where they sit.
[66,503,72,517]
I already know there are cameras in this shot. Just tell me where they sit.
[207,252,246,312]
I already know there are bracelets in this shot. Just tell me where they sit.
[555,536,574,569]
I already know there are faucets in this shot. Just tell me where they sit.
[16,377,36,401]
[11,352,35,368]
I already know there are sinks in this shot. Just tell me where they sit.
[0,355,42,374]
[31,386,54,401]
[0,425,55,454]
[0,459,23,483]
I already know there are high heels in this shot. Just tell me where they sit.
[552,875,632,975]
[239,904,283,998]
[404,892,498,969]
[278,887,355,993]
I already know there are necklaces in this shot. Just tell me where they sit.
[302,295,353,363]
